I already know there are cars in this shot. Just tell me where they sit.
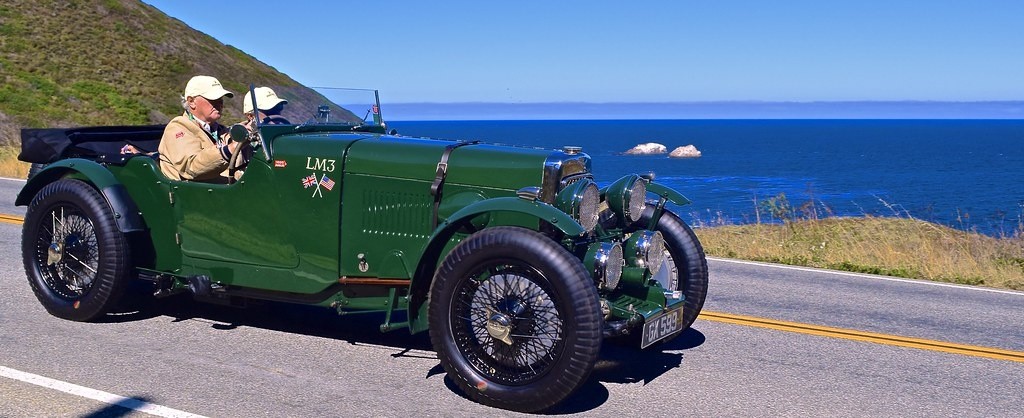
[12,75,710,413]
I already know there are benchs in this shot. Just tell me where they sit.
[50,121,230,167]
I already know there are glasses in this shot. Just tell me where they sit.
[256,102,284,115]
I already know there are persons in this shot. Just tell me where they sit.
[159,74,257,186]
[120,86,288,156]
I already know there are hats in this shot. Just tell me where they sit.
[184,76,234,100]
[243,87,288,115]
